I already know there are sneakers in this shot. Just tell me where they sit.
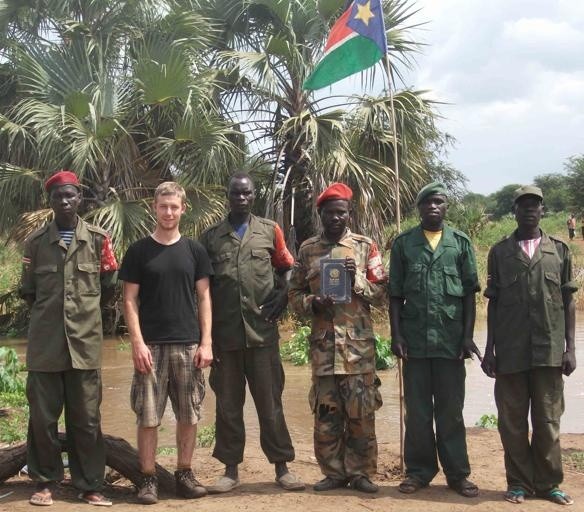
[174,468,208,498]
[137,473,158,504]
[274,462,306,491]
[207,464,241,492]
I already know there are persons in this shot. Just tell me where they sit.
[388,180,483,498]
[580,212,584,239]
[199,173,308,493]
[119,181,214,505]
[481,184,578,507]
[16,169,120,507]
[567,213,577,241]
[290,183,389,493]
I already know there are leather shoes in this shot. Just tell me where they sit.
[351,477,379,493]
[313,477,345,491]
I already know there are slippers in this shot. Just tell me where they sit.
[29,490,54,506]
[398,477,424,494]
[544,487,574,505]
[78,491,112,506]
[452,479,479,498]
[505,489,526,503]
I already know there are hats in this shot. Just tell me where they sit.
[44,171,79,191]
[415,182,448,206]
[512,185,544,203]
[316,182,353,207]
